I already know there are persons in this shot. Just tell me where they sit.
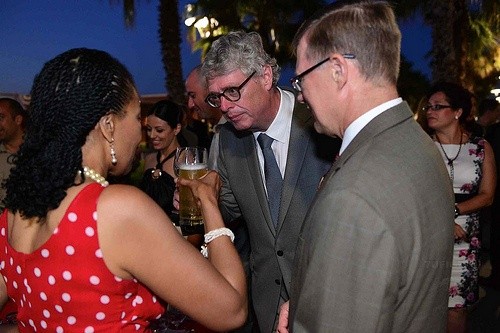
[424,84,497,333]
[141,100,189,224]
[173,30,342,333]
[0,98,28,215]
[0,47,247,333]
[186,65,228,133]
[276,0,455,333]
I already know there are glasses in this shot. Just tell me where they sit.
[290,54,355,92]
[423,105,452,111]
[204,70,257,108]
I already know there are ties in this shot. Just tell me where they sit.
[257,133,284,230]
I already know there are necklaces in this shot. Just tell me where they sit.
[434,131,463,166]
[78,166,109,187]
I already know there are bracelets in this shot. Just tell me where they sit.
[455,205,460,218]
[200,228,235,258]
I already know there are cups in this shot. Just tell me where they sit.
[175,146,207,235]
